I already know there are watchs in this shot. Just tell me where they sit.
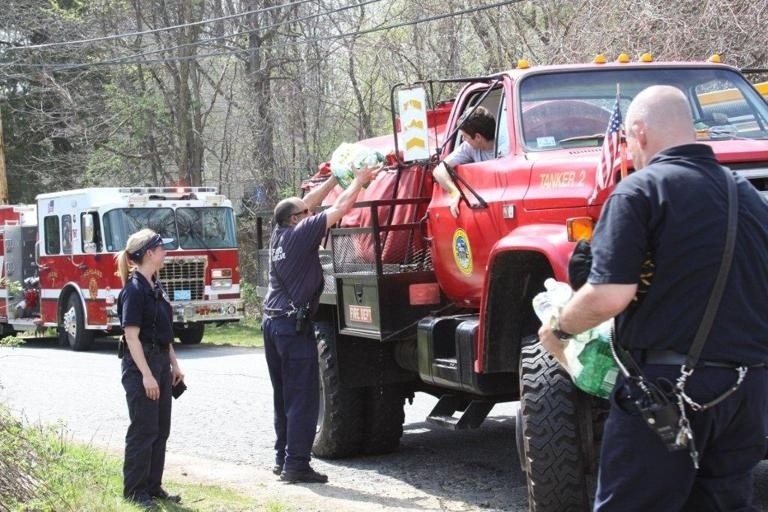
[549,308,575,342]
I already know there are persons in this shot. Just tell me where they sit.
[694,112,729,130]
[261,160,382,483]
[113,227,181,512]
[432,105,495,219]
[538,84,768,512]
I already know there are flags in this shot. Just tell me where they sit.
[602,101,622,189]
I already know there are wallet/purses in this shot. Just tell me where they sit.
[172,376,186,399]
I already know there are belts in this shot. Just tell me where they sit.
[644,352,766,371]
[264,309,282,315]
[160,348,170,351]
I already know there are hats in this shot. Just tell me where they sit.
[127,234,176,259]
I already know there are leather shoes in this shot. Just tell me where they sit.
[273,465,283,475]
[126,496,162,511]
[280,470,328,483]
[152,491,181,505]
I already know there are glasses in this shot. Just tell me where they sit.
[288,209,308,218]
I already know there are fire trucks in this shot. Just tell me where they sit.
[1,185,246,348]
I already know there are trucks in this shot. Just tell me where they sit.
[295,55,768,511]
